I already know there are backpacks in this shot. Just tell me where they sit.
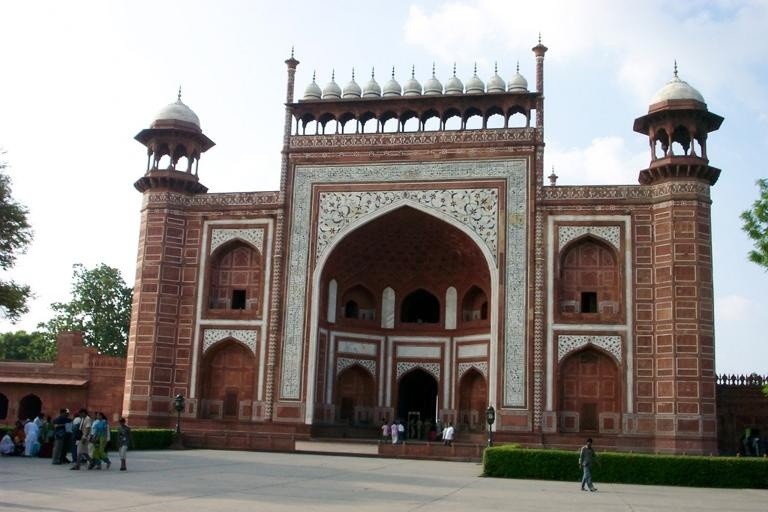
[1,434,15,454]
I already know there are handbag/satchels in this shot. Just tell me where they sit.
[55,430,65,439]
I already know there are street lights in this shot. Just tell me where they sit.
[174,394,184,432]
[487,405,495,447]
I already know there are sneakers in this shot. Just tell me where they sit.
[581,487,598,492]
[52,458,126,470]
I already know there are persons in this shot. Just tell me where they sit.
[381,418,433,445]
[441,422,448,447]
[444,422,454,447]
[576,439,598,492]
[0,408,131,473]
[734,431,767,458]
